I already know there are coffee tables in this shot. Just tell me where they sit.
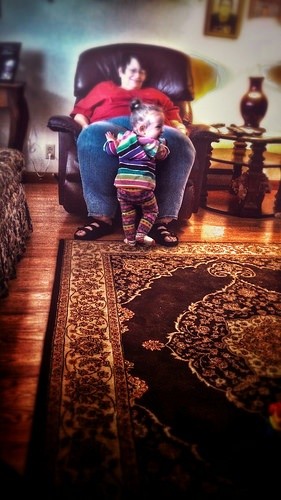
[200,124,281,220]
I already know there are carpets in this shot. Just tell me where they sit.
[27,238,281,500]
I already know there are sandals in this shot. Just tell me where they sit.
[151,223,179,247]
[74,220,113,240]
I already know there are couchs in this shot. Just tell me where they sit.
[47,43,220,223]
[1,148,33,298]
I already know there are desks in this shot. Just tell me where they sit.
[0,81,29,151]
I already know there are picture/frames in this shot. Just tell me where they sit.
[203,0,245,39]
[0,41,21,82]
[248,0,281,18]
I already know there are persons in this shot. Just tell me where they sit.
[105,98,170,248]
[70,52,196,247]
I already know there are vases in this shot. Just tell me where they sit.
[240,76,269,129]
